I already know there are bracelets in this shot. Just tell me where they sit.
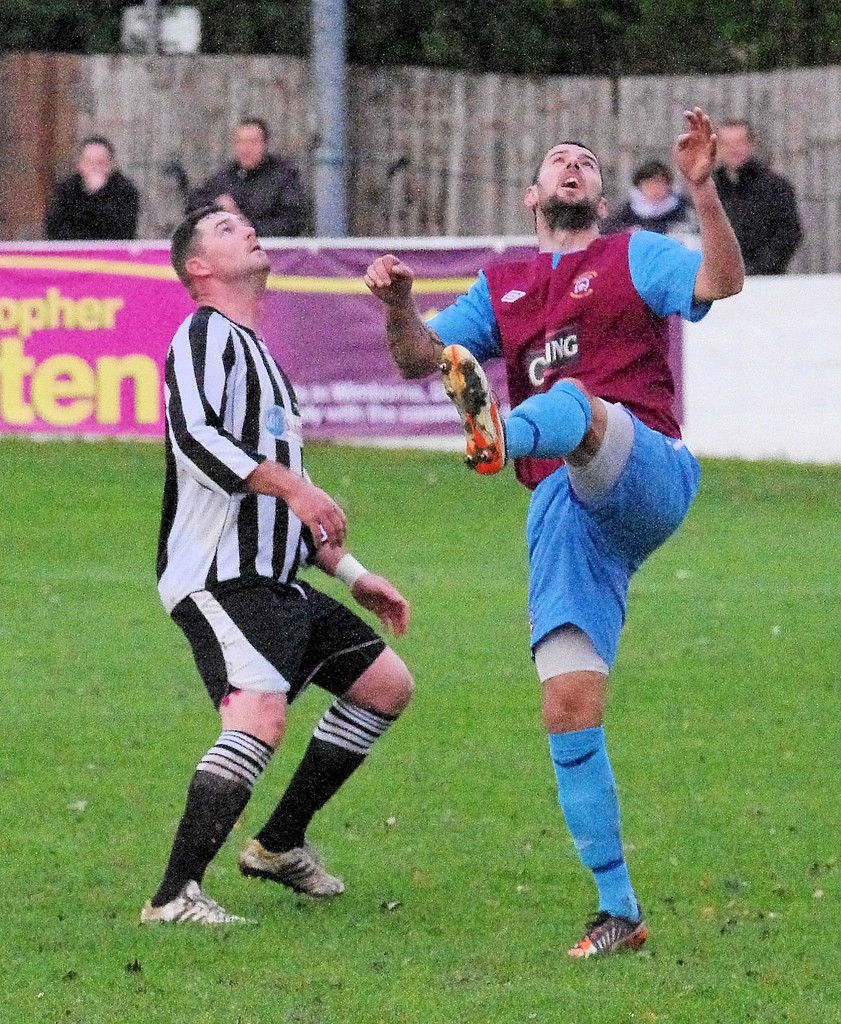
[335,554,364,585]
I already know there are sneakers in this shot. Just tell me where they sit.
[237,838,345,897]
[141,878,258,926]
[566,903,647,959]
[439,343,507,474]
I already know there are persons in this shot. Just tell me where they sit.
[362,107,744,961]
[46,137,138,241]
[712,117,804,274]
[138,204,415,926]
[186,116,315,235]
[609,160,699,235]
